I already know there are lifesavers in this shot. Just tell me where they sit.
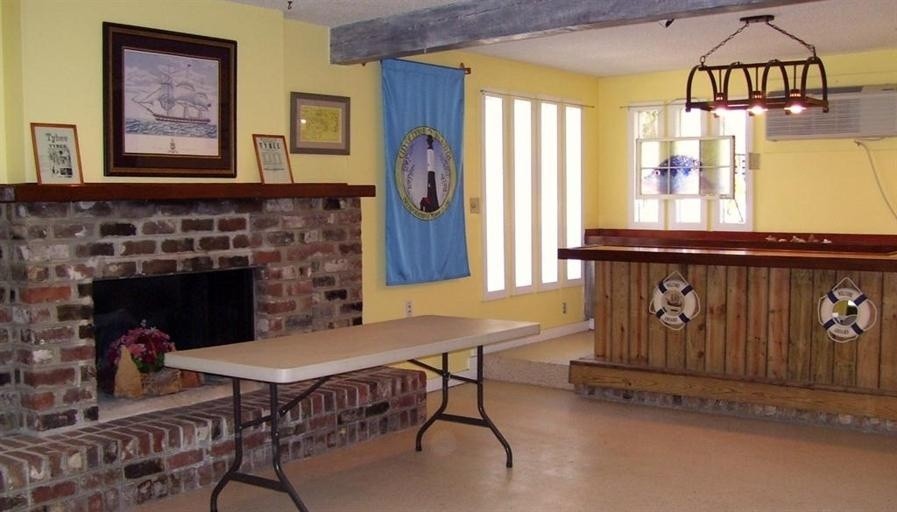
[650,280,696,325]
[820,287,872,337]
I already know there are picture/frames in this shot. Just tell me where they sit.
[100,19,239,180]
[250,133,292,183]
[636,135,737,201]
[29,121,82,186]
[289,89,352,157]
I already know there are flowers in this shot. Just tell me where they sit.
[104,316,178,375]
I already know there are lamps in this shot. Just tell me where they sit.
[684,16,829,117]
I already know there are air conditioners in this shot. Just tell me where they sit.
[765,84,897,143]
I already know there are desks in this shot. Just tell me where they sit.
[165,316,540,511]
